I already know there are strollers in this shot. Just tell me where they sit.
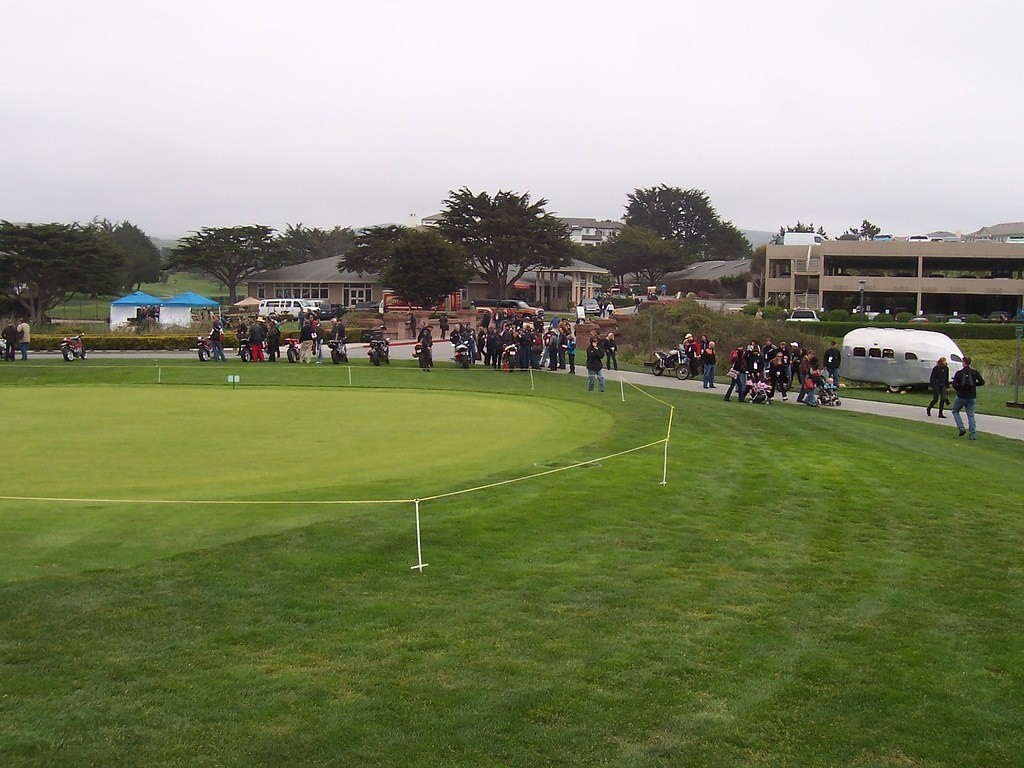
[814,375,841,406]
[746,369,772,406]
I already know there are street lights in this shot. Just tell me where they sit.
[858,280,867,322]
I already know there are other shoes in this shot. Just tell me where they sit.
[441,335,445,339]
[804,400,810,406]
[688,376,695,379]
[423,367,430,372]
[811,404,819,407]
[709,385,716,388]
[316,361,322,363]
[783,398,788,401]
[599,389,604,392]
[939,415,947,418]
[547,368,556,371]
[539,364,544,367]
[926,407,931,416]
[959,430,966,437]
[725,400,731,402]
[969,437,976,440]
[703,385,708,388]
[740,401,745,402]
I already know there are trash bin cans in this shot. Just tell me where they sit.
[361,329,383,343]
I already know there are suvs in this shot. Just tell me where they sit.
[497,300,545,322]
[320,304,349,321]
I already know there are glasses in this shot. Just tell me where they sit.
[593,340,598,342]
[777,356,782,357]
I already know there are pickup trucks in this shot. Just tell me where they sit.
[786,309,820,322]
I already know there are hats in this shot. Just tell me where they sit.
[685,334,692,338]
[790,342,798,348]
[550,328,557,335]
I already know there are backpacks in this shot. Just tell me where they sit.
[961,367,974,389]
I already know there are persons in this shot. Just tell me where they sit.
[235,312,280,362]
[756,308,764,320]
[414,323,433,372]
[683,331,717,389]
[450,308,600,374]
[209,315,227,362]
[407,312,418,340]
[598,299,616,319]
[1,321,17,362]
[439,313,449,339]
[587,337,605,392]
[927,357,950,419]
[135,303,161,331]
[952,357,985,440]
[603,332,618,370]
[633,283,667,313]
[723,336,846,409]
[17,318,31,360]
[299,307,347,364]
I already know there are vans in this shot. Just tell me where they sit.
[259,299,321,321]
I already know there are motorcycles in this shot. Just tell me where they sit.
[60,332,87,362]
[362,325,391,366]
[328,337,349,364]
[284,338,303,363]
[196,335,214,362]
[652,344,690,380]
[240,337,253,363]
[451,336,471,369]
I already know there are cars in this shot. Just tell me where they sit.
[783,232,1024,246]
[944,317,966,325]
[579,299,600,316]
[354,301,380,313]
[908,315,931,323]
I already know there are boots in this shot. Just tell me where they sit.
[797,392,804,403]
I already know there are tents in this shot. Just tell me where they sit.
[161,290,221,331]
[110,291,164,333]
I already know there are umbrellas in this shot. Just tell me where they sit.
[234,296,264,312]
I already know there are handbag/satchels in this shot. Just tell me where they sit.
[16,330,24,341]
[726,368,740,379]
